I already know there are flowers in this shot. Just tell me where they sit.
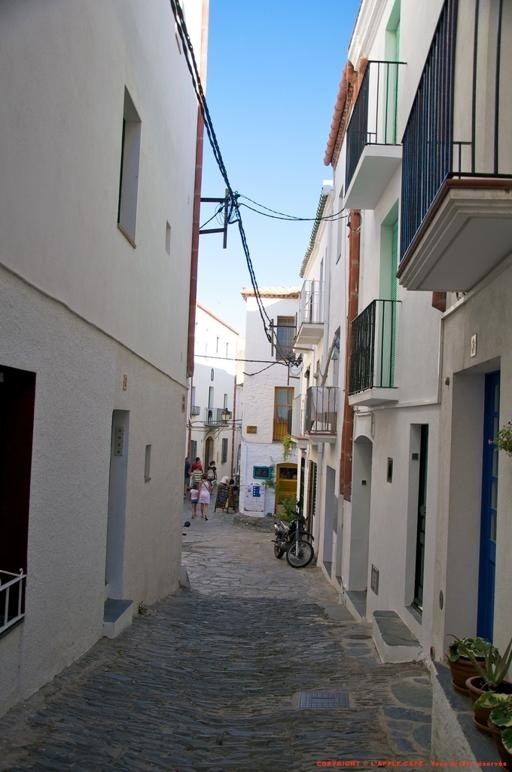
[487,418,512,457]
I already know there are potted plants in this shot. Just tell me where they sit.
[464,638,512,735]
[444,630,498,694]
[479,687,512,772]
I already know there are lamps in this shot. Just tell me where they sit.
[220,406,242,424]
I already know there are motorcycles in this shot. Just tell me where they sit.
[270,500,316,568]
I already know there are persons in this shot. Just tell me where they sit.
[184,457,193,498]
[188,483,199,519]
[207,461,218,495]
[199,474,214,520]
[191,456,203,482]
[214,478,229,512]
[229,478,236,486]
